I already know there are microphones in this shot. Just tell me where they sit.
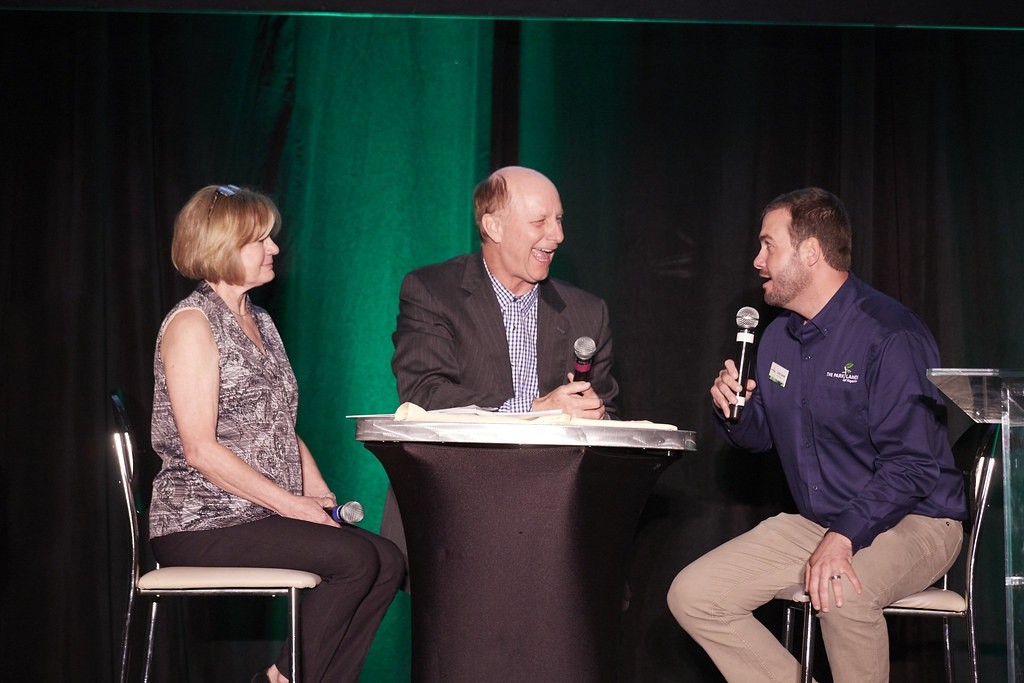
[570,336,596,398]
[729,307,760,419]
[323,500,365,525]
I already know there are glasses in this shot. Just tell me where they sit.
[207,183,242,224]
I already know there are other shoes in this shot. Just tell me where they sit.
[251,668,272,683]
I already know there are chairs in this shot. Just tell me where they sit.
[784,423,1001,683]
[110,394,322,683]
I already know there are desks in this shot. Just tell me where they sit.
[355,417,697,683]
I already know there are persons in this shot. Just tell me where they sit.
[378,165,620,597]
[148,185,406,683]
[666,188,969,683]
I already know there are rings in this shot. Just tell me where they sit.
[831,575,841,579]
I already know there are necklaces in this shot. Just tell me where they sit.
[230,310,249,316]
